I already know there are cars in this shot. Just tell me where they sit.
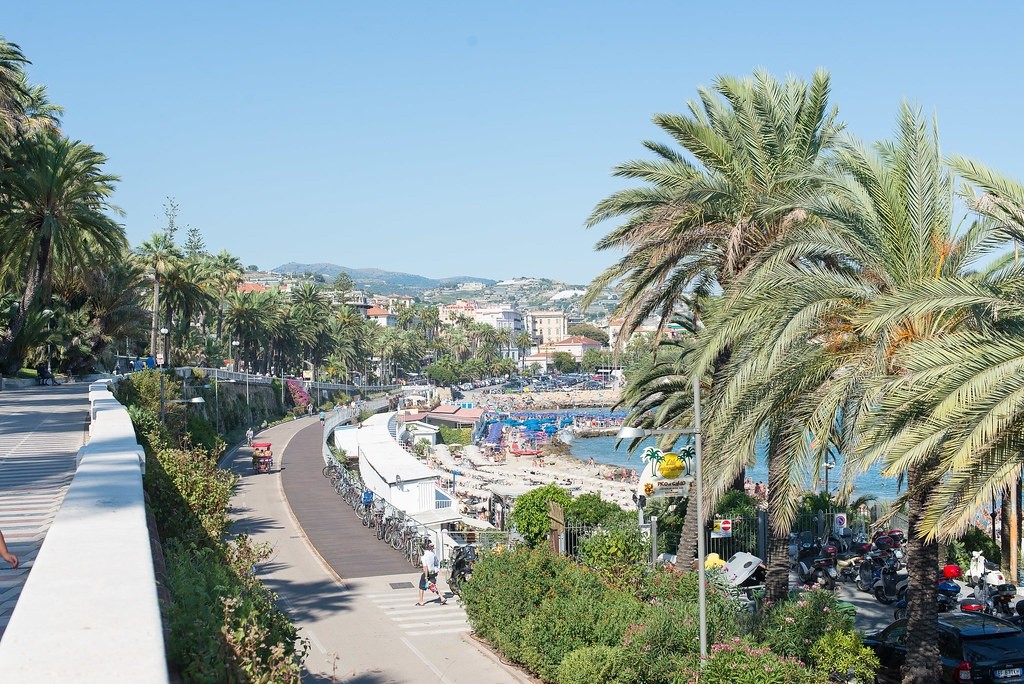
[857,610,1024,684]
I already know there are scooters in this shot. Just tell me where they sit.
[789,526,1024,629]
[444,543,482,599]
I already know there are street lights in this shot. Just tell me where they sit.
[232,340,241,372]
[147,271,158,358]
[160,328,169,365]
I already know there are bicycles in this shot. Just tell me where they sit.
[320,453,433,569]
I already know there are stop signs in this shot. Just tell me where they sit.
[722,521,732,533]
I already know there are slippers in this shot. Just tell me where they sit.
[414,602,425,607]
[440,599,447,605]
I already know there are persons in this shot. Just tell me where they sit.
[134,357,144,371]
[246,428,253,445]
[253,446,272,456]
[334,398,366,413]
[319,411,325,426]
[146,353,155,370]
[307,402,313,416]
[37,363,61,386]
[744,478,766,497]
[415,543,447,606]
[0,531,18,569]
